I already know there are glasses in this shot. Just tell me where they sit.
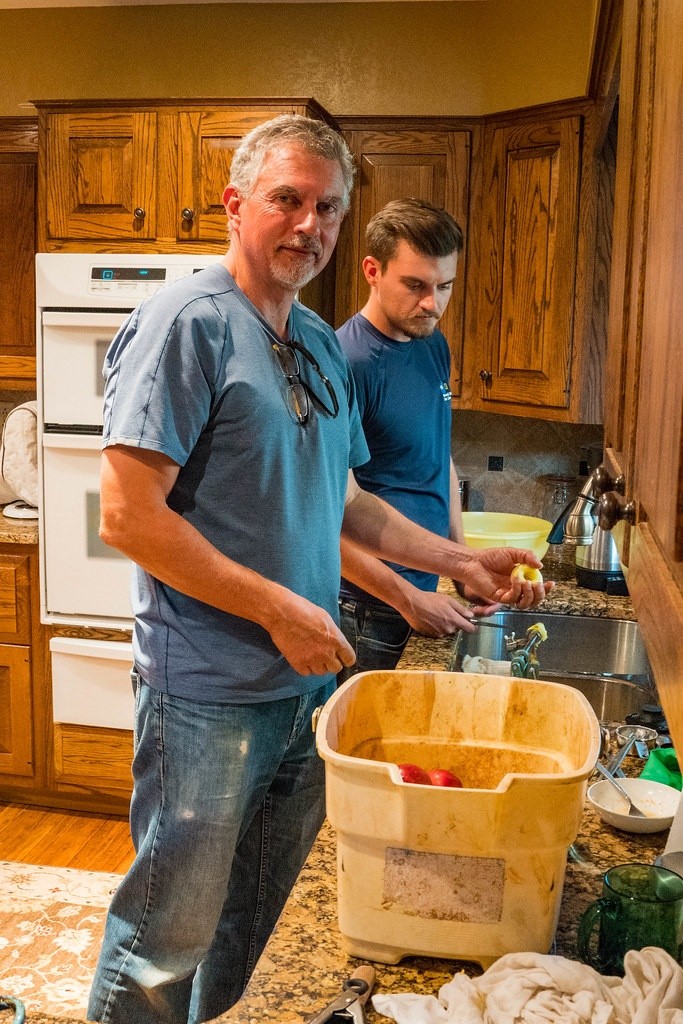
[272,344,310,428]
[286,340,339,419]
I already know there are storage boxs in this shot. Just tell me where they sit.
[311,668,601,976]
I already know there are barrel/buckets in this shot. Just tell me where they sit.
[312,668,603,974]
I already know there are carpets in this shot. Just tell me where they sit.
[0,860,126,1022]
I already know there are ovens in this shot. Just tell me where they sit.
[32,253,226,632]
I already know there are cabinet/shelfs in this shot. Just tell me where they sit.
[330,96,616,426]
[0,116,38,392]
[0,542,134,818]
[27,94,333,321]
[589,0,683,761]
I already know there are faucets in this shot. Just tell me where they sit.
[564,462,604,546]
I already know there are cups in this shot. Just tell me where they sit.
[576,862,683,980]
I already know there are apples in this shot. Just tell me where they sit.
[398,763,463,788]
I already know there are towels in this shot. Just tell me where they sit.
[369,944,683,1023]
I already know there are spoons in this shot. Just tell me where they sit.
[595,762,647,818]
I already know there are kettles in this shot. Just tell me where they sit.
[573,515,629,597]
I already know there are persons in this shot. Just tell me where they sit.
[88,113,554,1024]
[332,199,503,691]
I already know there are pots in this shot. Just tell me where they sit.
[615,725,659,760]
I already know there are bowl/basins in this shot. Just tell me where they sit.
[461,511,553,561]
[587,777,681,833]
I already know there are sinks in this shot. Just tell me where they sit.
[449,661,660,727]
[449,610,653,678]
